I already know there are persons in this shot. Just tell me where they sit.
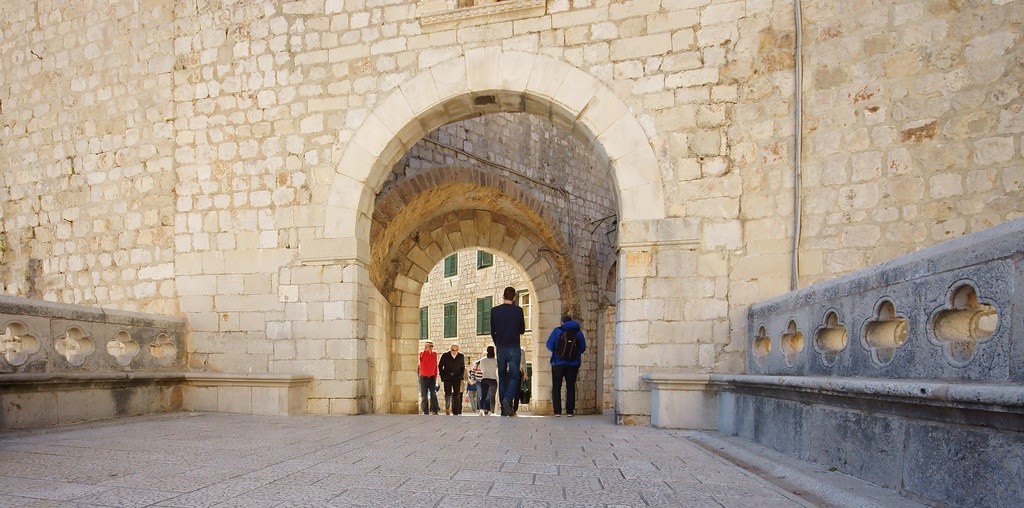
[546,315,586,417]
[418,286,528,417]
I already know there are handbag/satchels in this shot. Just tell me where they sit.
[520,381,530,404]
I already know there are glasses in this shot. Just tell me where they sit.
[452,349,457,351]
[429,346,433,347]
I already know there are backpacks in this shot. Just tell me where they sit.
[556,326,580,361]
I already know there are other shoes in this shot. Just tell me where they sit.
[479,409,484,416]
[453,411,458,415]
[503,397,514,416]
[567,410,574,417]
[555,412,561,417]
[432,412,440,416]
[500,407,506,415]
[423,410,429,415]
[446,409,450,415]
